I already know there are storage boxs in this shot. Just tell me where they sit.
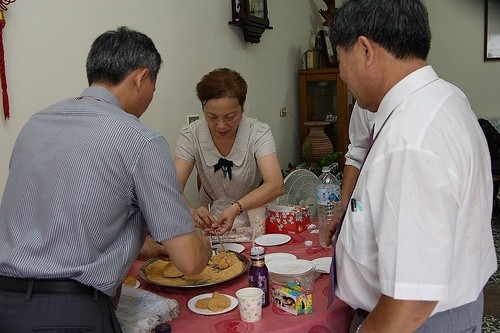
[270,281,313,316]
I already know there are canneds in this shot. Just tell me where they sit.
[307,48,320,70]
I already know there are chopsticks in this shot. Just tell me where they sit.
[208,203,226,254]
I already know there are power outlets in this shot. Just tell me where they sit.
[280,107,287,117]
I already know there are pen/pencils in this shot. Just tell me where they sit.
[351,198,356,212]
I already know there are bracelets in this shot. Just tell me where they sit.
[231,200,243,217]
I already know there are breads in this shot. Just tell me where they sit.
[195,290,230,312]
[163,248,242,283]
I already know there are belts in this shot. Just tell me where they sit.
[0,277,109,297]
[355,308,370,319]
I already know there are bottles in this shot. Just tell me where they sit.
[248,246,270,307]
[317,166,340,248]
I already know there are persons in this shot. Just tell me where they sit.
[326,0,497,333]
[325,99,381,246]
[0,26,211,333]
[173,67,285,236]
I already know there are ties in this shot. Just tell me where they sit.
[327,123,375,310]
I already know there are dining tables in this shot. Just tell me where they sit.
[123,218,354,333]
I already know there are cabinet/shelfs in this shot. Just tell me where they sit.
[298,67,355,170]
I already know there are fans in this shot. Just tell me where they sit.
[275,169,321,222]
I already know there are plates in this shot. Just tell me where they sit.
[254,233,291,246]
[312,256,334,274]
[269,259,312,275]
[139,248,250,289]
[186,292,238,315]
[212,243,244,254]
[126,276,140,288]
[265,253,297,263]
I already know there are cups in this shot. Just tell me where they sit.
[301,47,316,68]
[235,287,264,323]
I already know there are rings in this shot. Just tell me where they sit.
[198,219,201,223]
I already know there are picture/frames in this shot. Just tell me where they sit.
[483,0,500,61]
[228,0,274,43]
